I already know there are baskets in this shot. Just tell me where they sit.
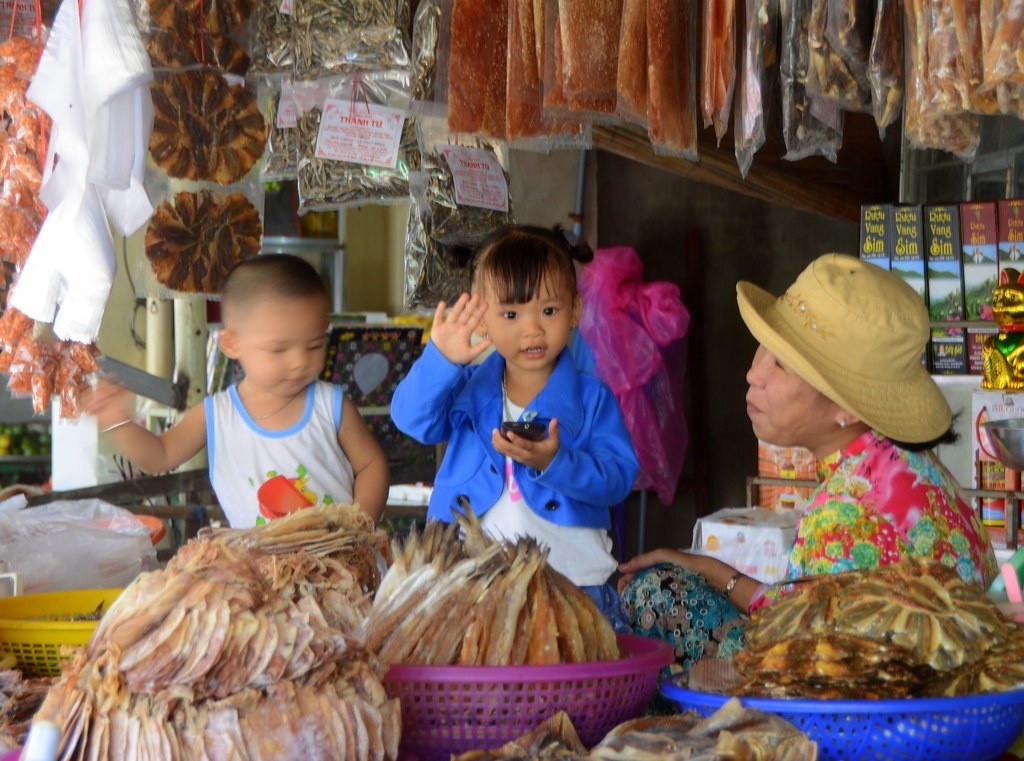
[381,633,675,761]
[0,587,123,678]
[658,670,1023,761]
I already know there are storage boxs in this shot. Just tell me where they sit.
[859,204,890,272]
[959,202,999,375]
[971,386,1024,557]
[997,200,1024,288]
[889,203,928,308]
[698,505,803,584]
[923,203,966,375]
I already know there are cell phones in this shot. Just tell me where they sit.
[501,421,546,441]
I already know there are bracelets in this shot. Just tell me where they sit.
[103,417,133,435]
[724,574,737,599]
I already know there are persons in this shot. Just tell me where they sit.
[616,249,999,629]
[387,216,642,614]
[81,254,388,539]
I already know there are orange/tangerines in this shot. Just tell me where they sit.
[0,422,52,457]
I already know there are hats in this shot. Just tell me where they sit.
[737,251,954,443]
